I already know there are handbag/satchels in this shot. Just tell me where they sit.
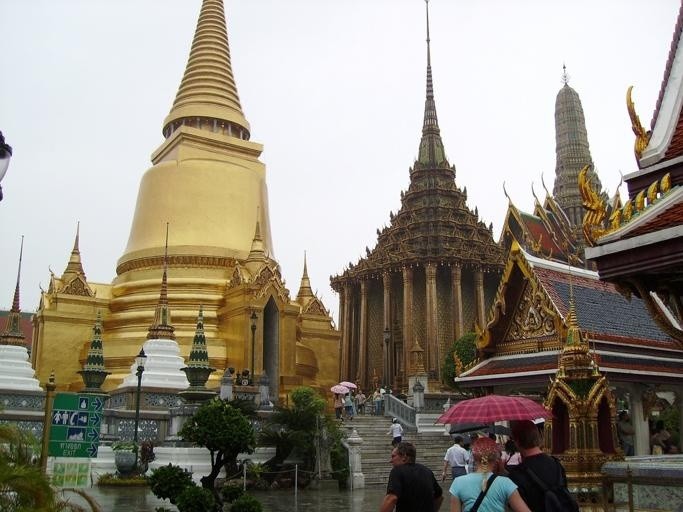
[341,397,345,404]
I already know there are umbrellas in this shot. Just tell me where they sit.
[434,394,553,435]
[331,381,358,394]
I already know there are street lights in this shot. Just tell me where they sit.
[384,328,390,394]
[134,348,147,442]
[250,311,258,385]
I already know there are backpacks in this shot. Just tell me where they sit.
[529,469,578,512]
[397,467,434,511]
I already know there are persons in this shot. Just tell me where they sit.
[333,389,367,420]
[442,420,568,512]
[649,416,673,455]
[373,384,393,415]
[617,411,636,456]
[387,417,404,444]
[237,369,253,386]
[379,441,444,512]
